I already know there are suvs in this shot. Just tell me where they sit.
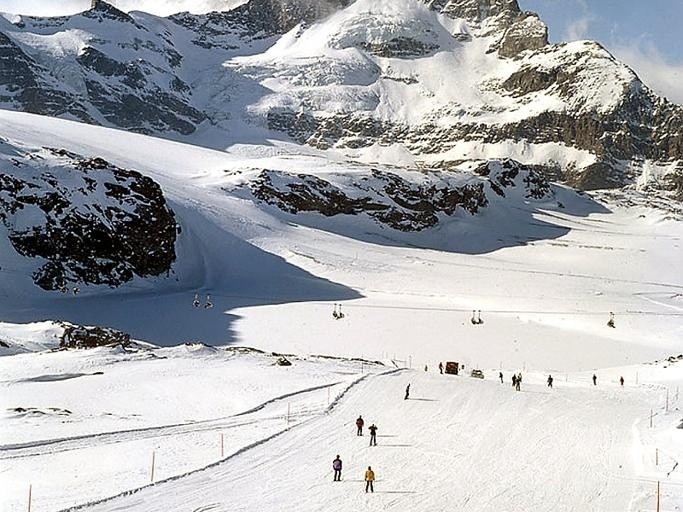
[471,369,484,379]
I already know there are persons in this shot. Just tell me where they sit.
[403,383,410,400]
[619,376,623,385]
[498,372,522,392]
[592,374,597,385]
[546,374,553,387]
[354,415,363,436]
[368,423,377,446]
[364,465,374,493]
[332,454,342,481]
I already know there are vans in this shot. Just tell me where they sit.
[445,360,458,375]
